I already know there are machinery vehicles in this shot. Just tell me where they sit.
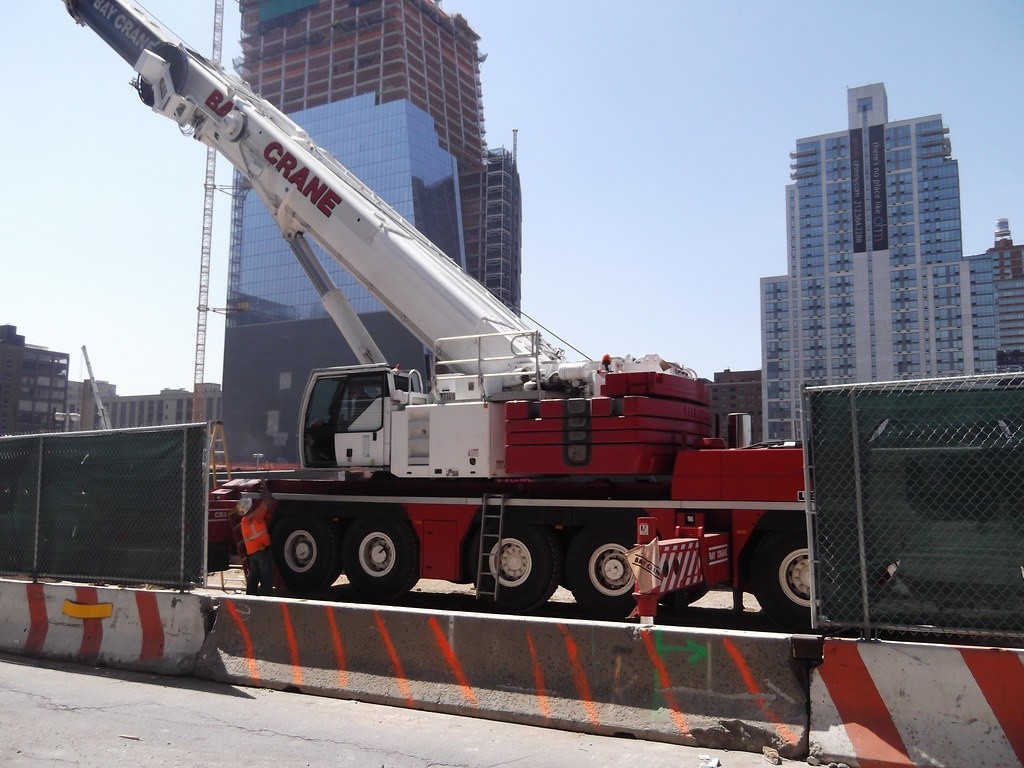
[64,0,823,627]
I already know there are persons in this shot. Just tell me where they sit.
[318,384,373,460]
[237,475,274,596]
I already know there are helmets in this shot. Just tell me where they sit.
[237,498,252,515]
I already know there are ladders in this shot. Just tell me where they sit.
[209,420,232,490]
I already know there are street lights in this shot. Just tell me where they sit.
[253,454,264,472]
[55,411,81,432]
